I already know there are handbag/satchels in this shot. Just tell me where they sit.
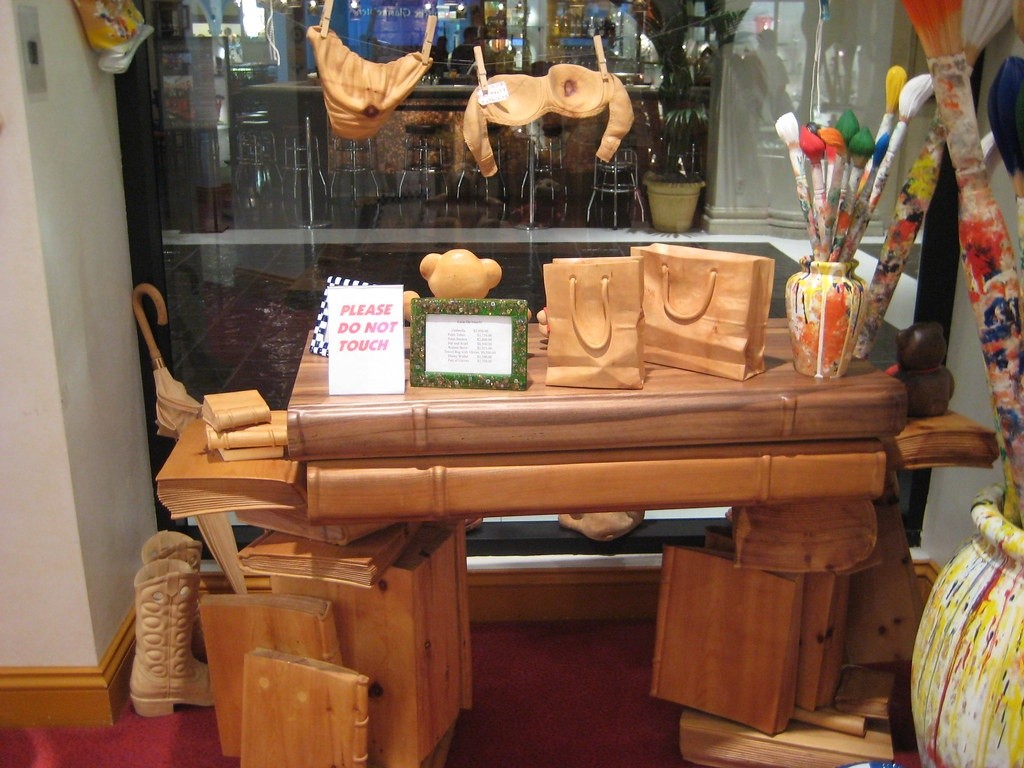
[630,242,774,382]
[541,255,646,389]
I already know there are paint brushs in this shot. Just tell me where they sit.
[774,65,935,266]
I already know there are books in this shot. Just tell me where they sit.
[156,390,420,588]
[650,414,997,768]
[199,594,367,768]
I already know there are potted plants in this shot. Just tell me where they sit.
[638,1,726,234]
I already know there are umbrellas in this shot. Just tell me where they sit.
[132,284,246,595]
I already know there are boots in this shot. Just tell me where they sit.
[141,528,211,660]
[127,560,216,716]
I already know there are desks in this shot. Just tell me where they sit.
[270,316,909,767]
